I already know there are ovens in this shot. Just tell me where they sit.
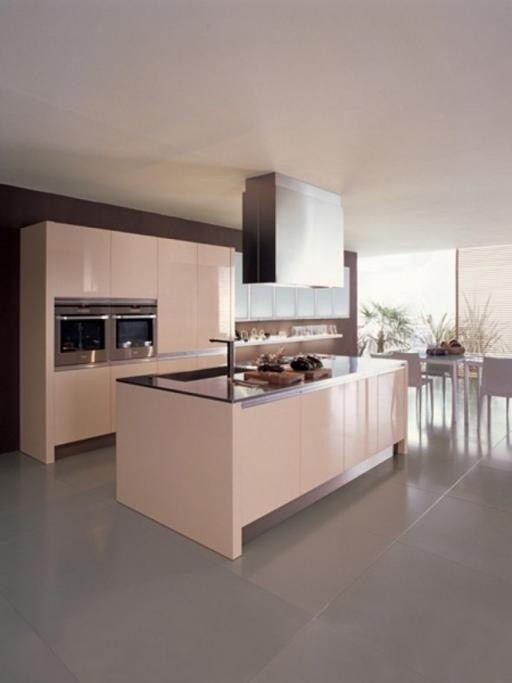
[53,300,158,365]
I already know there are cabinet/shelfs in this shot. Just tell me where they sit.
[234,252,350,321]
[20,220,158,300]
[18,353,228,463]
[157,236,235,356]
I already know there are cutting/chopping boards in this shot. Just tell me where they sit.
[244,371,304,388]
[280,367,332,382]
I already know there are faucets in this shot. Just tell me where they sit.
[209,337,235,378]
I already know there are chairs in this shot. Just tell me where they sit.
[371,351,433,431]
[476,355,512,435]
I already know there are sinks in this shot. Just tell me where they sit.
[155,365,257,381]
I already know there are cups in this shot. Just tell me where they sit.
[292,324,337,335]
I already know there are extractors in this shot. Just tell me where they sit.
[241,172,345,288]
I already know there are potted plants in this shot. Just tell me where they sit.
[419,311,457,355]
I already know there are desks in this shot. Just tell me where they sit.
[420,351,482,433]
